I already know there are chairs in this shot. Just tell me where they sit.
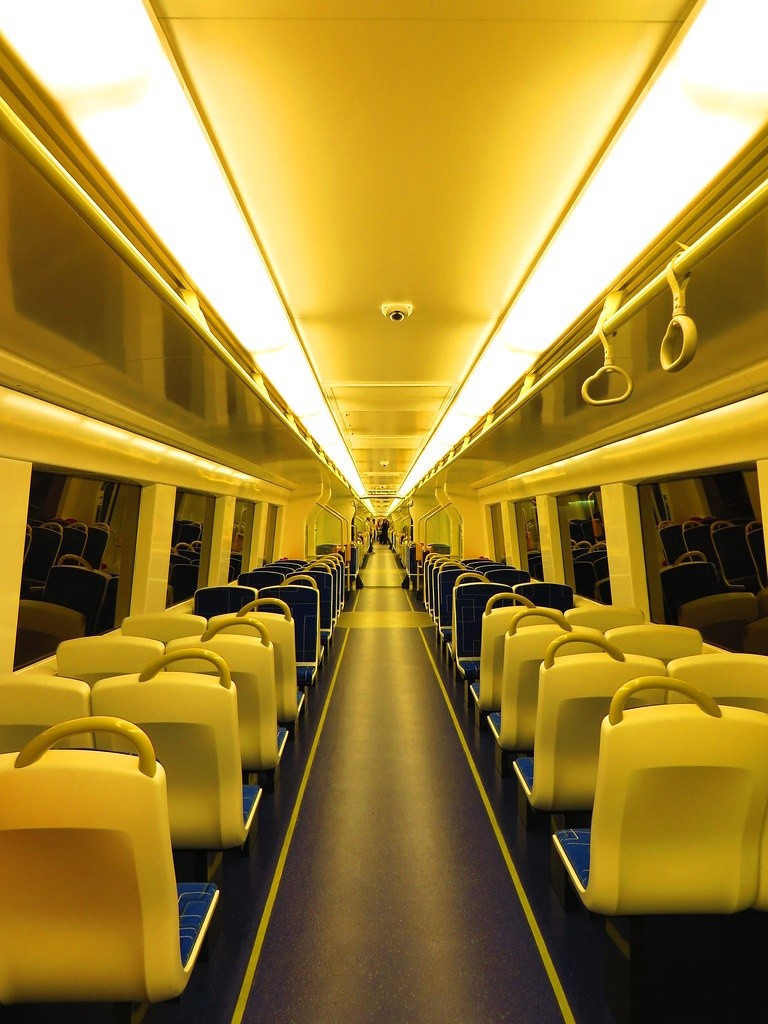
[165,520,203,608]
[656,515,768,654]
[12,518,122,671]
[0,542,360,1024]
[568,511,612,606]
[424,553,768,1024]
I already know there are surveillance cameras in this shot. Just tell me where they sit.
[380,461,389,467]
[382,303,414,321]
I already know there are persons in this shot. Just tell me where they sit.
[363,516,389,545]
